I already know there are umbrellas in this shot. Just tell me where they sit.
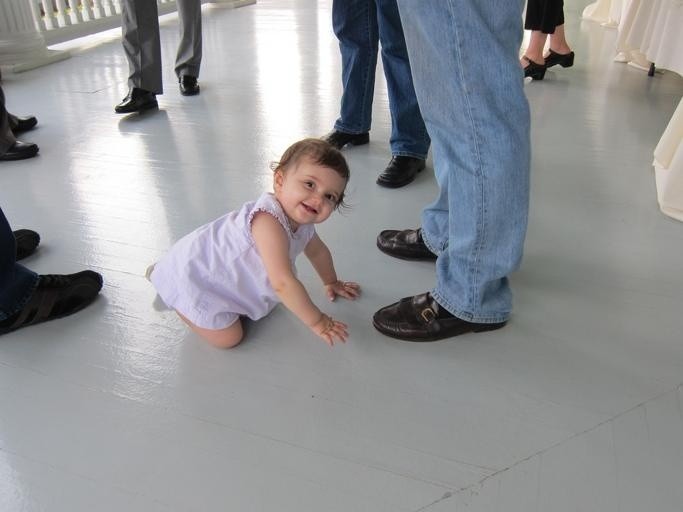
[0,271,103,334]
[115,88,158,113]
[12,229,40,262]
[320,129,369,150]
[376,228,437,261]
[0,115,39,161]
[179,76,199,95]
[376,156,425,188]
[373,292,506,342]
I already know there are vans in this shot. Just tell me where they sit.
[523,48,574,80]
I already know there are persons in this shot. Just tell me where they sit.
[113,0,202,113]
[320,0,430,190]
[372,1,530,342]
[0,83,39,162]
[520,0,574,80]
[0,208,103,338]
[143,137,361,349]
